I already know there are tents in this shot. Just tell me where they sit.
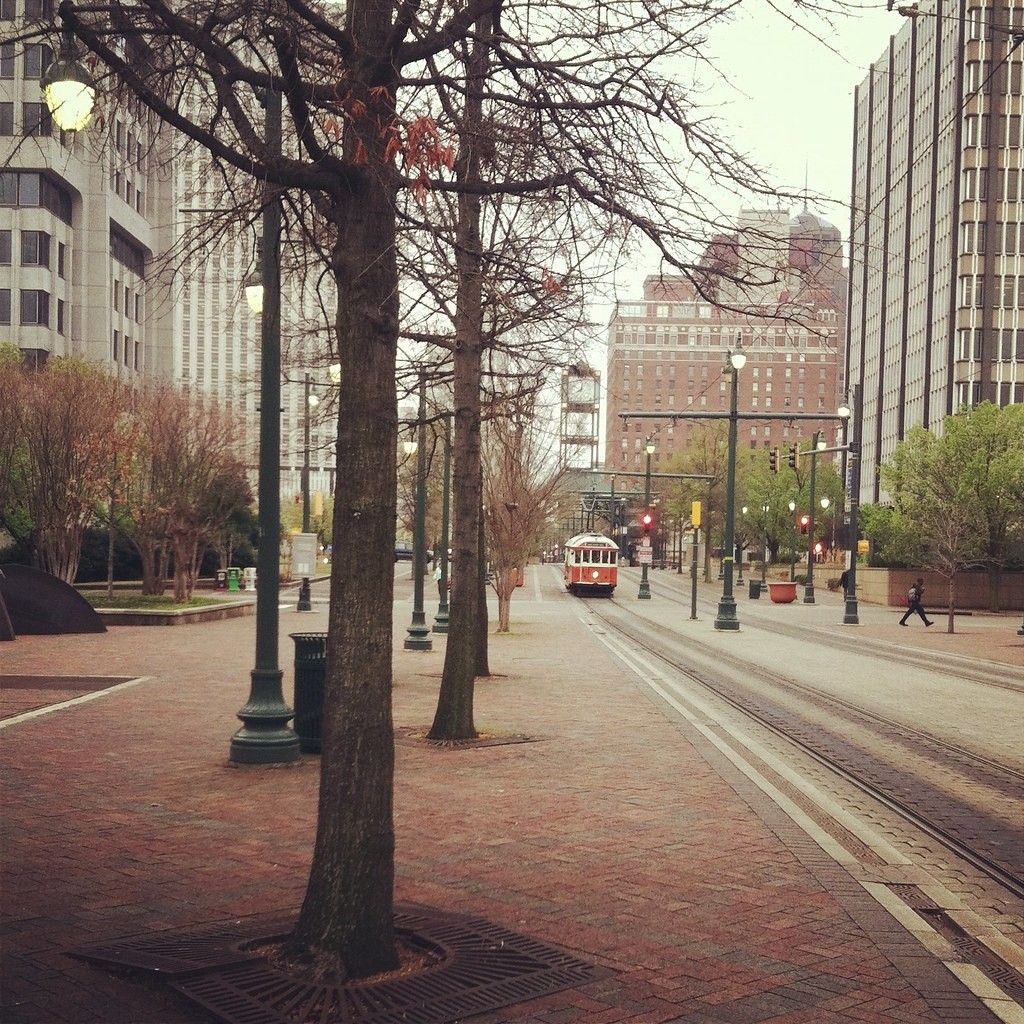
[0,563,109,641]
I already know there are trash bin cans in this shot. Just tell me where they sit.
[289,631,328,752]
[749,578,763,599]
[216,570,227,592]
[228,567,240,592]
[242,567,257,591]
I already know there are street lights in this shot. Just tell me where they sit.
[787,497,798,582]
[39,0,301,766]
[431,407,452,634]
[637,435,656,600]
[759,500,770,593]
[819,494,835,563]
[735,505,748,587]
[401,361,434,651]
[714,331,748,630]
[296,372,320,611]
[803,429,828,604]
[837,402,851,521]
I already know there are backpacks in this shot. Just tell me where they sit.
[908,587,921,602]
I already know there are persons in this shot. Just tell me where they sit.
[628,542,636,567]
[839,570,849,601]
[434,563,441,593]
[899,578,934,627]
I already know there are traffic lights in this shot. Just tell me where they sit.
[788,445,801,470]
[768,446,780,474]
[814,542,823,554]
[799,513,811,535]
[642,514,653,534]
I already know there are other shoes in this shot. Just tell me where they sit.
[899,621,909,626]
[925,622,934,627]
